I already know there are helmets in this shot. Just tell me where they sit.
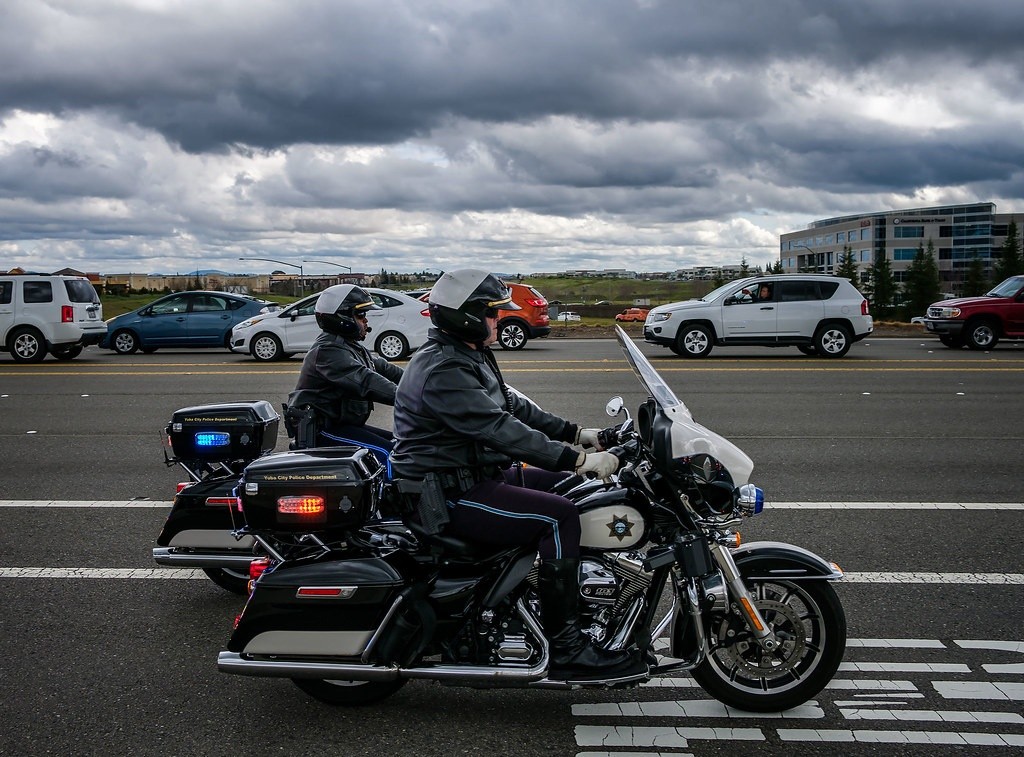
[313,283,383,342]
[428,269,524,343]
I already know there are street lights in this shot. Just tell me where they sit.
[302,260,353,285]
[240,257,305,297]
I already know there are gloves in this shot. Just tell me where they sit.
[575,425,607,452]
[574,451,620,480]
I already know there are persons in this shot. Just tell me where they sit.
[388,268,647,682]
[741,284,771,303]
[282,284,406,485]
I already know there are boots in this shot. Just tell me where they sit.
[536,554,644,681]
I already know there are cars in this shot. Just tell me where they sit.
[228,286,436,362]
[615,308,649,321]
[557,312,581,322]
[99,290,280,355]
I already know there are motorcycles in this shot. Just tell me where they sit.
[153,395,394,600]
[219,321,850,713]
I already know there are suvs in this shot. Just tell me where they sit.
[0,274,108,364]
[642,274,873,359]
[923,274,1024,350]
[419,281,551,351]
